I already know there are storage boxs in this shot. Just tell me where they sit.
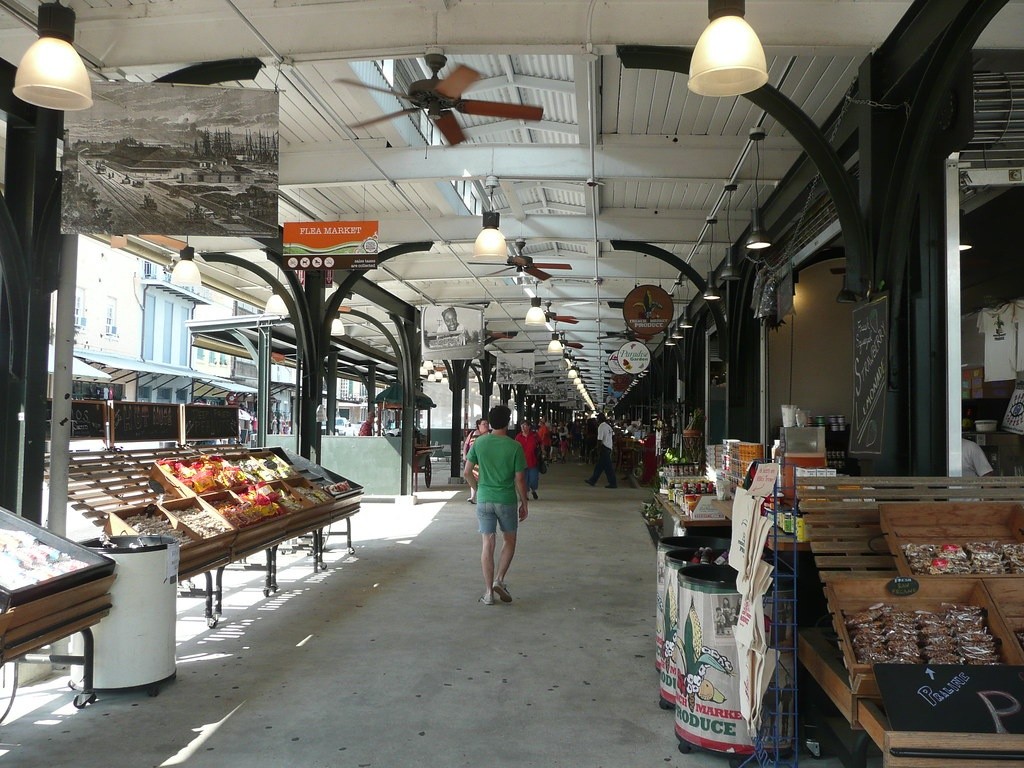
[0,448,337,664]
[690,494,726,519]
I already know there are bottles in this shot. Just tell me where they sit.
[701,555,709,564]
[713,548,730,565]
[771,439,781,463]
[762,497,815,543]
[667,477,715,518]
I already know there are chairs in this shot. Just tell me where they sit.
[441,444,451,465]
[612,432,637,474]
[429,441,438,457]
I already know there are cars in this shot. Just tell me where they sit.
[320,418,351,437]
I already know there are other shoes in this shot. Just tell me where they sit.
[467,498,477,504]
[605,485,617,489]
[584,479,594,486]
[532,491,538,499]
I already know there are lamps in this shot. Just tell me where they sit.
[331,311,345,337]
[524,283,547,327]
[567,365,577,378]
[170,234,203,288]
[580,388,585,393]
[11,3,93,111]
[836,275,858,303]
[264,265,289,316]
[560,351,572,370]
[573,377,581,385]
[472,212,508,261]
[665,0,769,347]
[576,383,583,389]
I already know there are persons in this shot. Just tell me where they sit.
[583,412,617,489]
[963,439,993,502]
[436,307,467,345]
[271,416,291,435]
[358,412,375,436]
[530,417,598,468]
[462,418,491,504]
[515,420,540,500]
[464,405,528,605]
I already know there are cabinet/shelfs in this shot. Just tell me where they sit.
[962,430,1024,476]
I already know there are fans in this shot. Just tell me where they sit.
[322,61,599,414]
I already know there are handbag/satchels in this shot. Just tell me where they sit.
[532,432,547,474]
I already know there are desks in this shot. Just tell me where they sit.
[712,499,847,625]
[654,491,732,537]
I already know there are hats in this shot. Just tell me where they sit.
[595,413,605,418]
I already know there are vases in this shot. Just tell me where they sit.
[683,429,702,451]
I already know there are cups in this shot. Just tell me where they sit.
[975,420,997,433]
[781,404,811,429]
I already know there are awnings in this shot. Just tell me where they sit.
[191,379,258,403]
[71,350,234,394]
[269,381,302,396]
[48,344,112,383]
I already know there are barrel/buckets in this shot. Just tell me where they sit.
[654,536,797,768]
[67,534,181,698]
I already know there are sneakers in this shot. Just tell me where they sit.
[492,580,512,602]
[481,595,493,605]
